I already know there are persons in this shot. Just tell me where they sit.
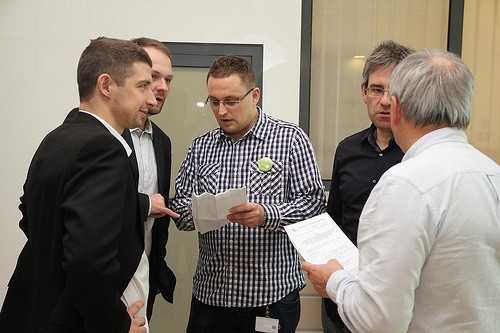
[168,54,328,333]
[298,39,500,333]
[0,36,181,333]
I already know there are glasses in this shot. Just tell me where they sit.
[364,87,390,98]
[205,88,254,107]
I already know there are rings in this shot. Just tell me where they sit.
[299,262,303,270]
